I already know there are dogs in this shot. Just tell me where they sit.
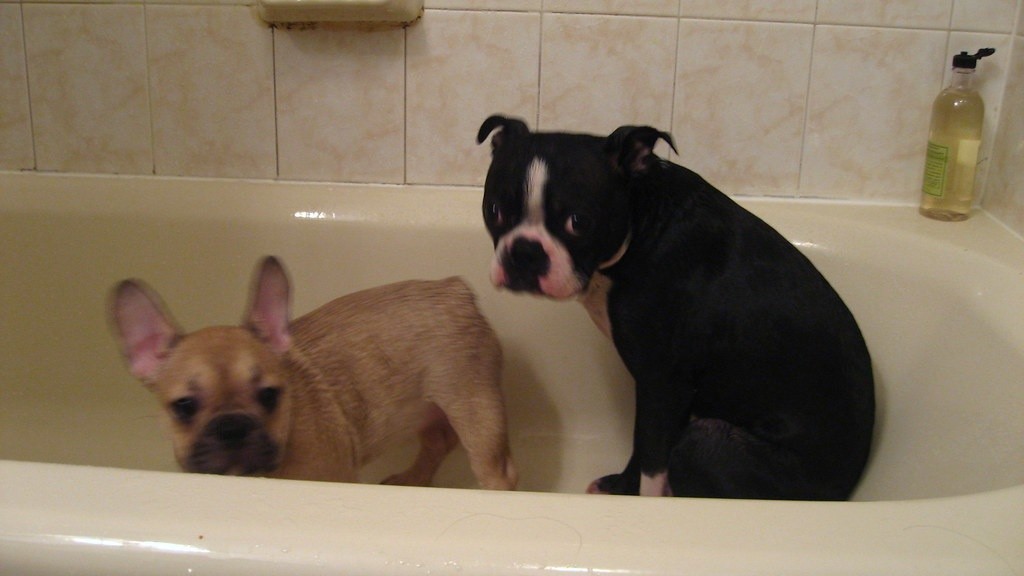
[108,255,520,493]
[475,113,876,503]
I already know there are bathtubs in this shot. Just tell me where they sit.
[1,168,1024,576]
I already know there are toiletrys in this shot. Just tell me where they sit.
[918,46,997,224]
